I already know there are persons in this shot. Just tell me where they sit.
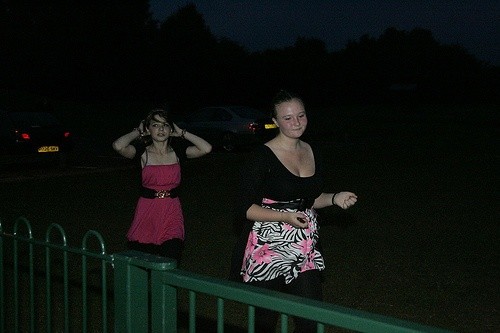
[234,90,359,333]
[112,108,212,275]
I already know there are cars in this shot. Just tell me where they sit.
[177,106,278,153]
[0,110,76,165]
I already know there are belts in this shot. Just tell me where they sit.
[140,186,179,199]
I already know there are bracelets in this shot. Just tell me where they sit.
[330,190,339,206]
[132,127,144,140]
[181,127,188,138]
